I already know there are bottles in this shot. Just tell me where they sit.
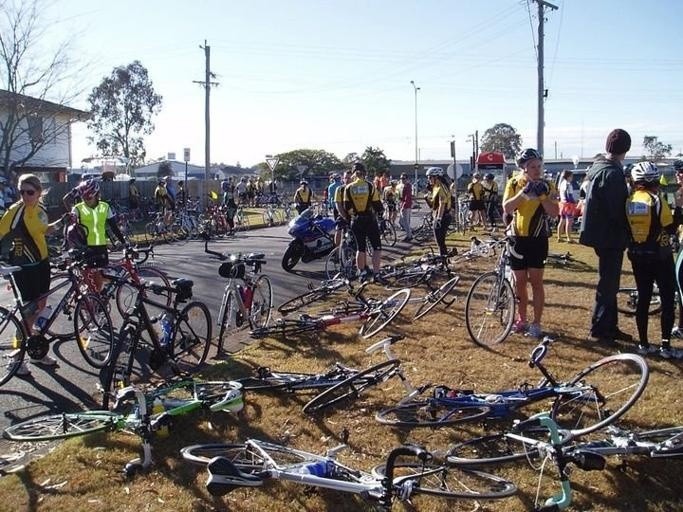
[161,315,171,335]
[31,304,52,331]
[242,286,252,308]
[484,394,503,403]
[152,396,166,414]
[236,284,244,302]
[299,460,333,475]
[585,440,606,447]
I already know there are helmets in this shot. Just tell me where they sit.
[515,148,544,168]
[78,179,100,199]
[631,161,660,184]
[426,167,444,176]
[352,163,365,173]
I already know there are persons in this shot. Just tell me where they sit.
[450,171,499,227]
[424,166,450,253]
[1,174,77,370]
[503,128,682,359]
[220,176,277,236]
[293,180,318,217]
[128,177,187,222]
[324,164,413,284]
[62,173,131,328]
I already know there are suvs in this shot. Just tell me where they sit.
[554,167,589,204]
[652,161,678,185]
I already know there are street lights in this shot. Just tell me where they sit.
[410,80,424,194]
[449,134,458,228]
[464,134,476,166]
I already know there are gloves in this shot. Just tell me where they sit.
[522,180,550,197]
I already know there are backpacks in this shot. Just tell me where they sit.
[62,221,89,252]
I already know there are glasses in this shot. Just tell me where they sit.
[19,190,37,196]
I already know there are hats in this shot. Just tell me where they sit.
[606,129,632,155]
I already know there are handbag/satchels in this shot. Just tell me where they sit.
[1,228,42,267]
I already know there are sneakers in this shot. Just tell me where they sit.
[390,226,413,242]
[510,319,542,338]
[30,353,57,365]
[358,271,390,285]
[470,225,499,233]
[637,326,683,358]
[6,358,31,375]
[557,238,577,244]
